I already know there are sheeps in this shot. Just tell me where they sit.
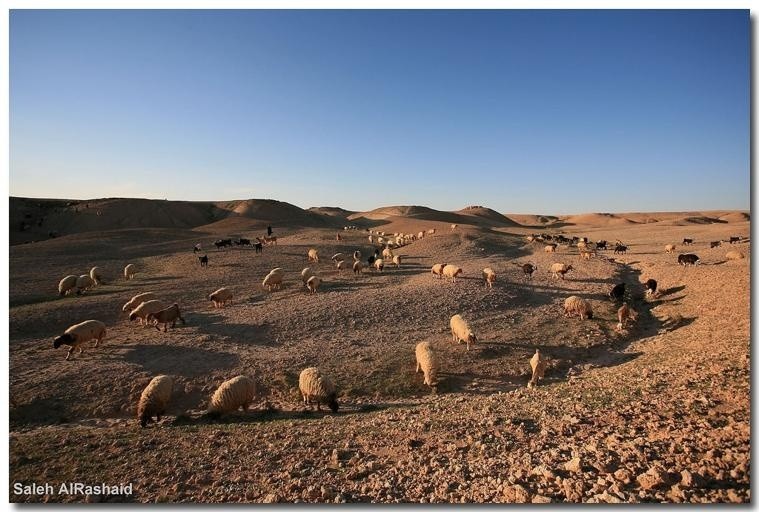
[297,365,341,417]
[205,374,257,420]
[51,223,751,362]
[136,373,176,429]
[416,340,441,393]
[527,349,548,390]
